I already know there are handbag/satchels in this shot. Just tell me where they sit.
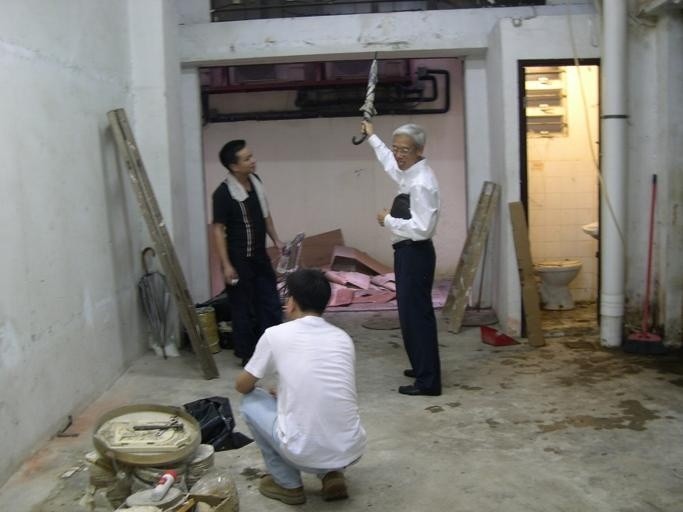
[391,193,412,220]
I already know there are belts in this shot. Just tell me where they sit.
[392,239,433,250]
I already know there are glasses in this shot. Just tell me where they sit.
[391,145,410,154]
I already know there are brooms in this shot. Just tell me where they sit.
[622,174,669,355]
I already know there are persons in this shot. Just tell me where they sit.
[235,268,366,505]
[360,120,443,397]
[211,140,289,366]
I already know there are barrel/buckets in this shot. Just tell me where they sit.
[193,306,222,355]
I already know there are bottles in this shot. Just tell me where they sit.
[150,470,176,501]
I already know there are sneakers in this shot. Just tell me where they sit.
[320,471,349,501]
[259,474,306,505]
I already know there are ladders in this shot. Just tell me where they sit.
[107,107,219,380]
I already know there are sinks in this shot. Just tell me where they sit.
[582,222,600,240]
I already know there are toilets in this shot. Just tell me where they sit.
[532,260,583,311]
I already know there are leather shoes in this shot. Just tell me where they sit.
[399,369,442,396]
